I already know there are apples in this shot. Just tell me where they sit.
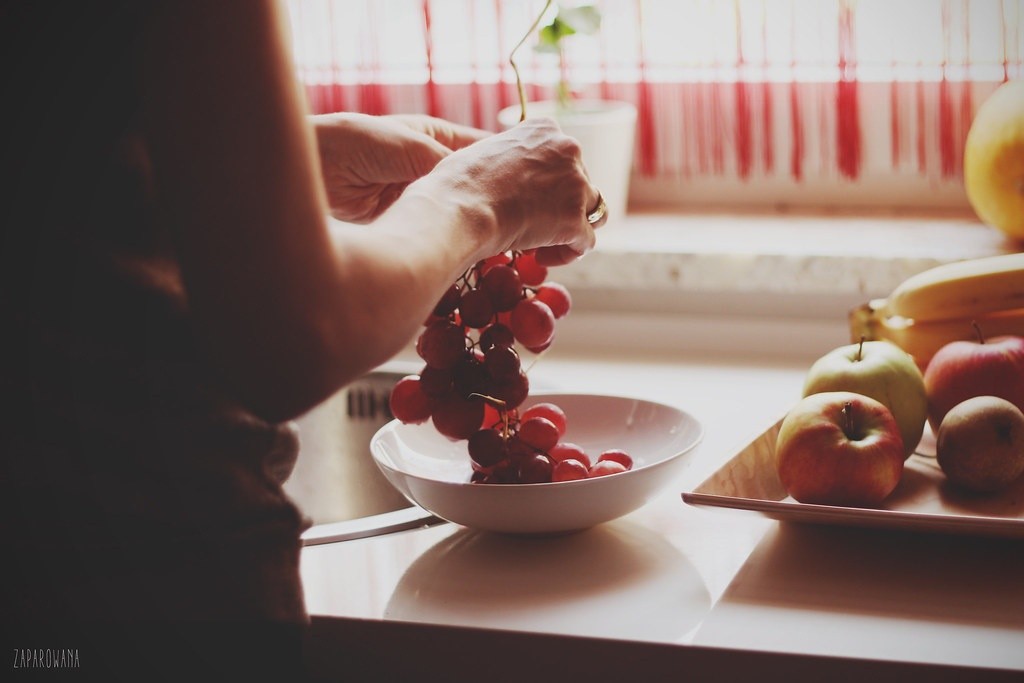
[925,320,1024,437]
[936,397,1024,488]
[803,335,927,460]
[963,75,1024,242]
[776,391,905,506]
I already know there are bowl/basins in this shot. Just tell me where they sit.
[369,393,705,537]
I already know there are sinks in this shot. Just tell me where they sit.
[280,364,452,546]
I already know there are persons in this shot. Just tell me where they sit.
[1,0,611,682]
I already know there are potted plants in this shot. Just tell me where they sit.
[496,1,636,223]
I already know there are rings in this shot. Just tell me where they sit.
[586,187,606,223]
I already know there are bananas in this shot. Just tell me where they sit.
[848,252,1024,367]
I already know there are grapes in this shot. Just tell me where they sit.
[389,246,634,484]
